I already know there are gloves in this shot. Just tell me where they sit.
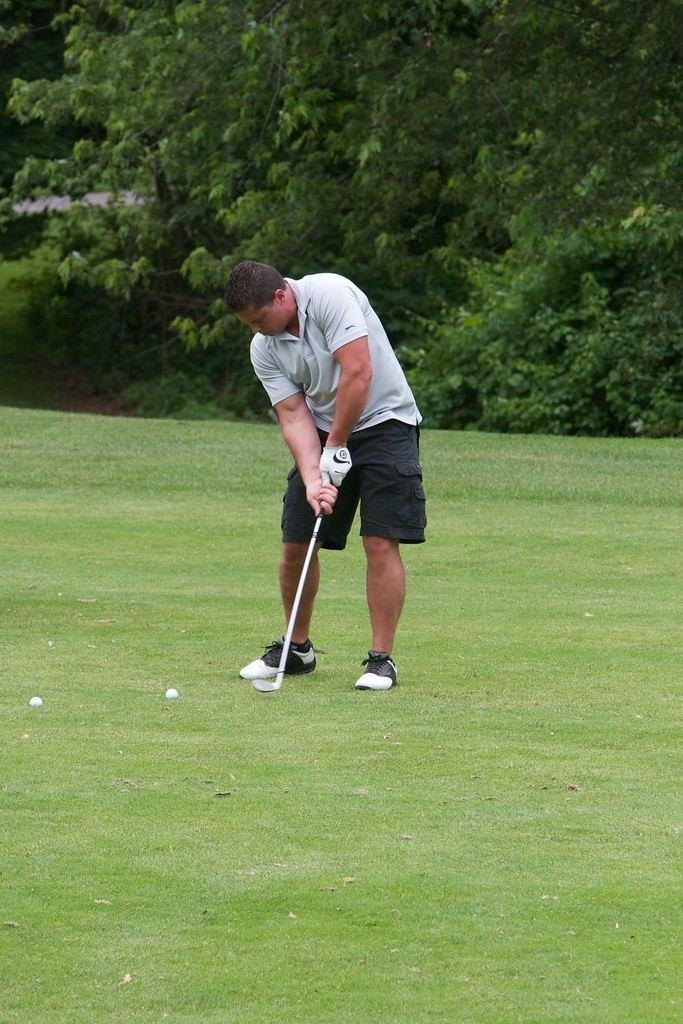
[318,443,353,488]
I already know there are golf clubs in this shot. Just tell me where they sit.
[251,509,326,694]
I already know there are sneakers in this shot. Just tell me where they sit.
[239,641,317,681]
[352,650,398,691]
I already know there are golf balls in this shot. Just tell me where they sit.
[164,689,178,698]
[29,697,43,706]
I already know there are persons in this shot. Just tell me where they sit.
[224,262,427,690]
